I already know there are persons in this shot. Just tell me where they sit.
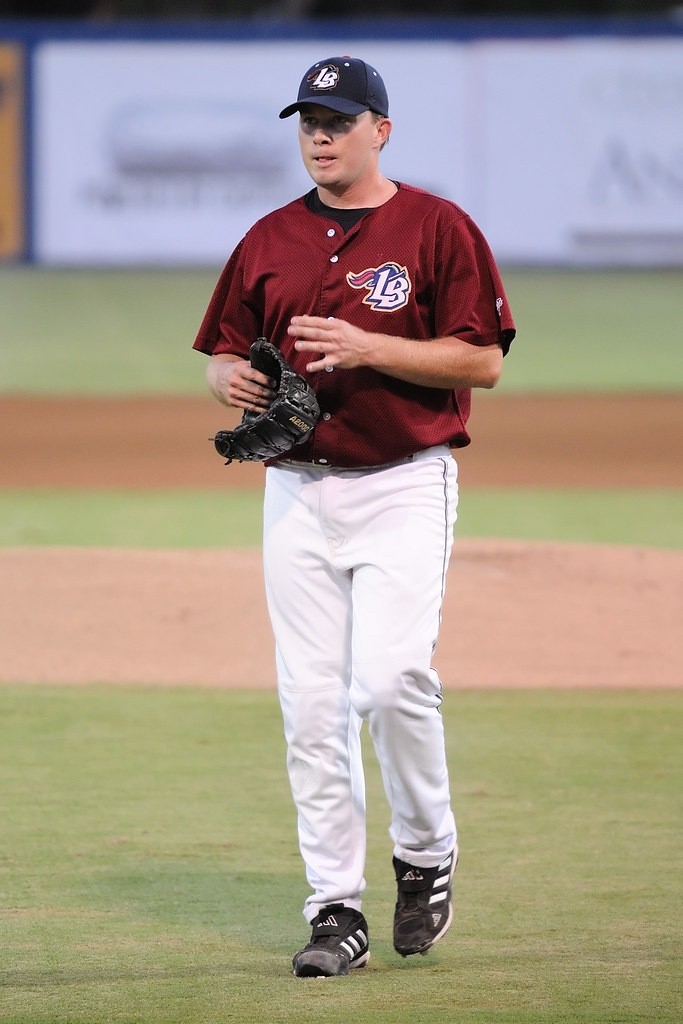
[192,54,514,976]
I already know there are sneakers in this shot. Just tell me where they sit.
[392,844,459,956]
[292,903,371,977]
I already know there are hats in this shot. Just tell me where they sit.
[279,55,390,119]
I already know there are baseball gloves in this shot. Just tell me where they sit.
[212,336,323,464]
